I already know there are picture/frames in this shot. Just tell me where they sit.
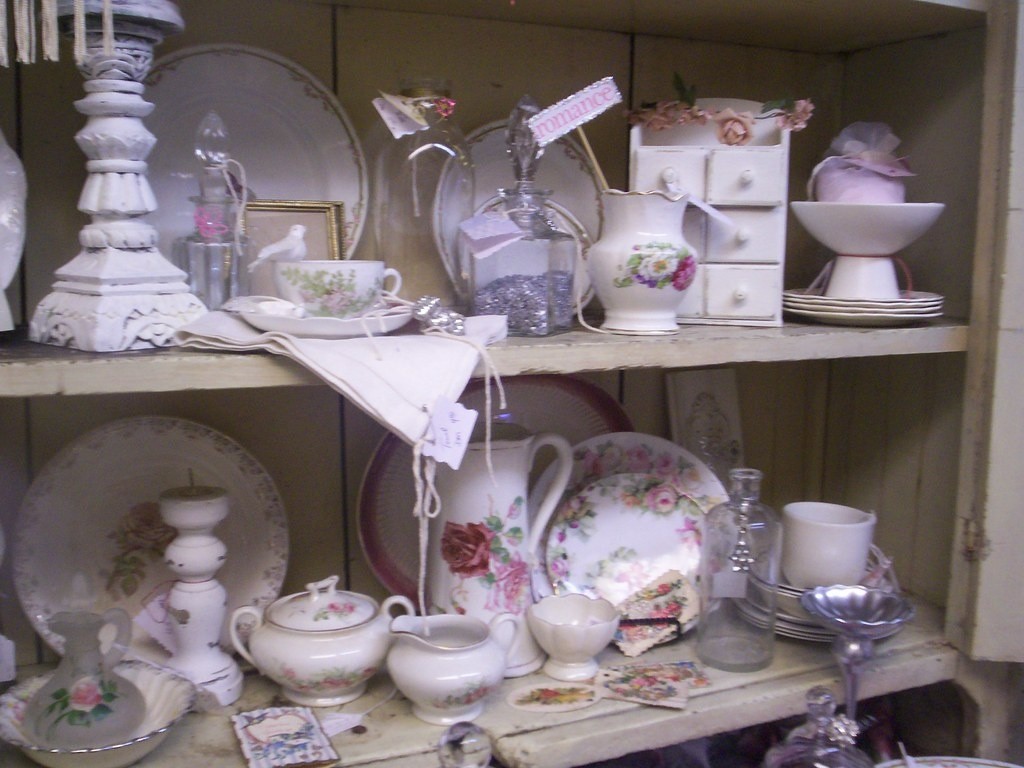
[238,198,347,297]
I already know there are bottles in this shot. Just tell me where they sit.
[451,93,576,336]
[585,189,700,336]
[698,468,783,674]
[171,113,251,310]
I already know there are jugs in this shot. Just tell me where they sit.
[386,610,521,726]
[24,607,146,751]
[427,420,573,680]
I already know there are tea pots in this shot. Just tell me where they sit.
[9,416,289,663]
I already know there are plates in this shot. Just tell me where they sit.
[736,590,905,643]
[354,372,635,618]
[240,297,416,340]
[873,755,1023,768]
[544,472,707,642]
[459,196,598,316]
[141,43,370,303]
[529,430,730,643]
[782,287,945,326]
[430,120,606,283]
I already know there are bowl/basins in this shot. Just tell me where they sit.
[790,201,946,256]
[0,660,198,768]
[822,255,900,300]
[746,542,890,621]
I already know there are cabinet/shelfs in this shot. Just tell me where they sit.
[0,0,1023,768]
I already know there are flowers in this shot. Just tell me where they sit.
[619,94,814,146]
[429,96,456,117]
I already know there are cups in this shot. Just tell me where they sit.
[526,594,620,682]
[270,260,403,318]
[783,501,877,590]
